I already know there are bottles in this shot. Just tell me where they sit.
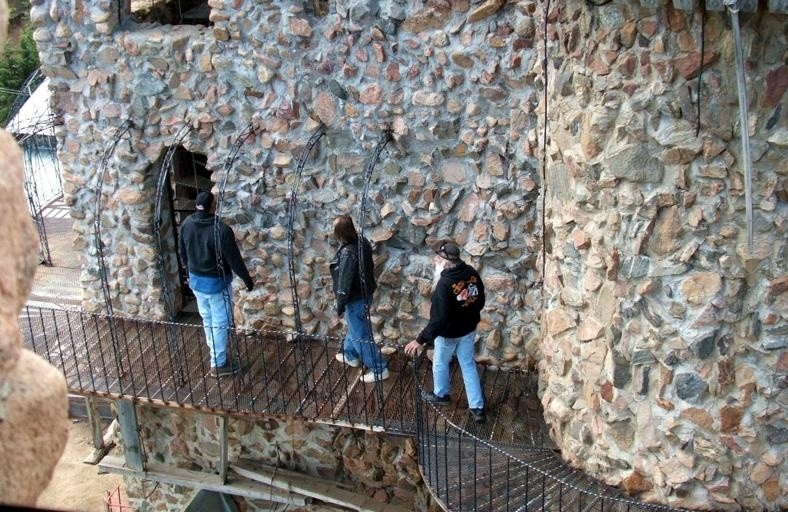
[435,242,462,264]
[195,191,213,218]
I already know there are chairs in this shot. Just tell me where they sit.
[359,368,389,383]
[210,364,239,378]
[469,408,483,422]
[420,391,451,406]
[335,353,360,368]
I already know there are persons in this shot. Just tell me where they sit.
[403,240,486,425]
[178,191,253,379]
[326,214,391,383]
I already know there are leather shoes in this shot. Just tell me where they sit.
[243,276,253,292]
